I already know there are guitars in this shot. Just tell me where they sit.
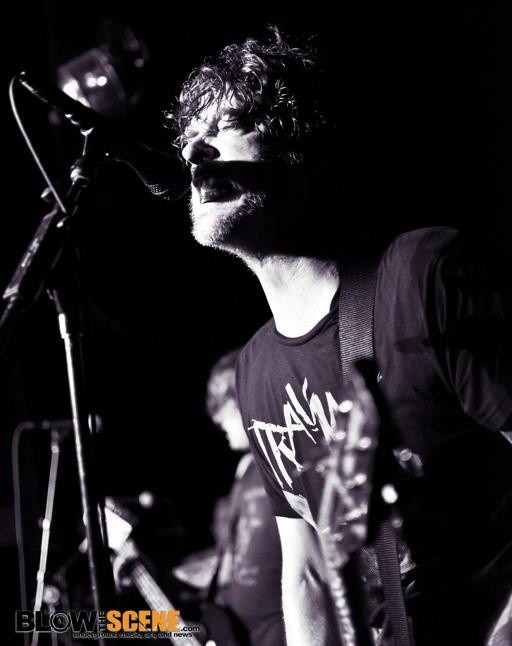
[79,494,252,646]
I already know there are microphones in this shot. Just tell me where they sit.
[39,409,107,439]
[18,68,186,204]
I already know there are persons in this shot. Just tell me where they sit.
[202,348,286,645]
[163,18,511,645]
[159,496,245,645]
